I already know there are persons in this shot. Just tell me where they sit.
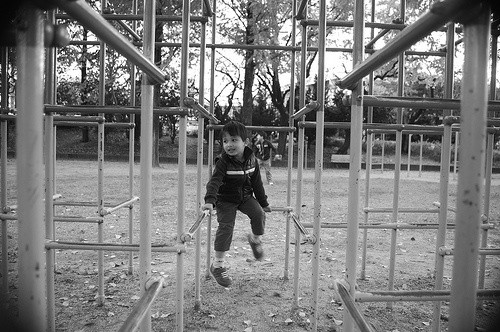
[202,122,271,286]
[252,133,281,185]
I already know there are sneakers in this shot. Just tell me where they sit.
[247,233,266,260]
[209,263,233,288]
[268,182,273,186]
